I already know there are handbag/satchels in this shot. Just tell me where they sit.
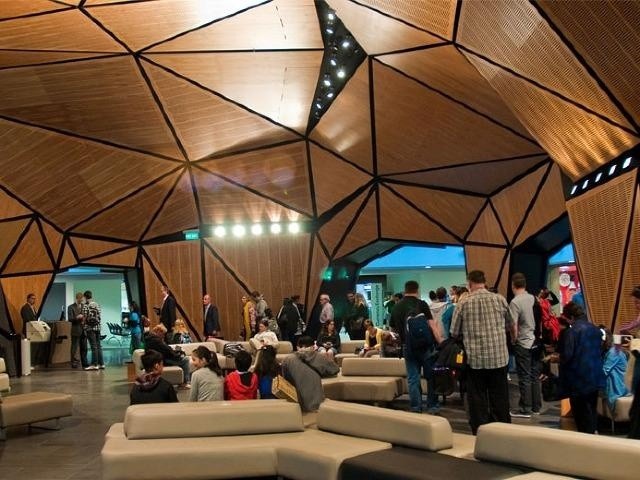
[271,374,298,403]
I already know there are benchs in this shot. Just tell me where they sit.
[0,390,73,437]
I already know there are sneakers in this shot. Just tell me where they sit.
[509,409,539,418]
[82,364,105,370]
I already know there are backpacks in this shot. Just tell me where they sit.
[404,308,431,359]
[86,308,101,326]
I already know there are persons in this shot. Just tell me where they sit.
[559,301,628,434]
[351,293,370,340]
[383,269,570,434]
[361,320,397,357]
[68,292,90,369]
[343,292,355,339]
[20,293,39,338]
[80,290,106,371]
[124,283,342,414]
[621,286,640,439]
